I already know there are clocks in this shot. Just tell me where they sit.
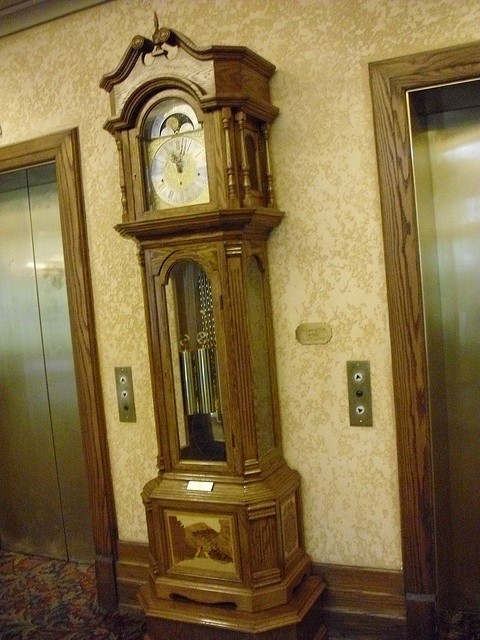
[100,11,328,640]
